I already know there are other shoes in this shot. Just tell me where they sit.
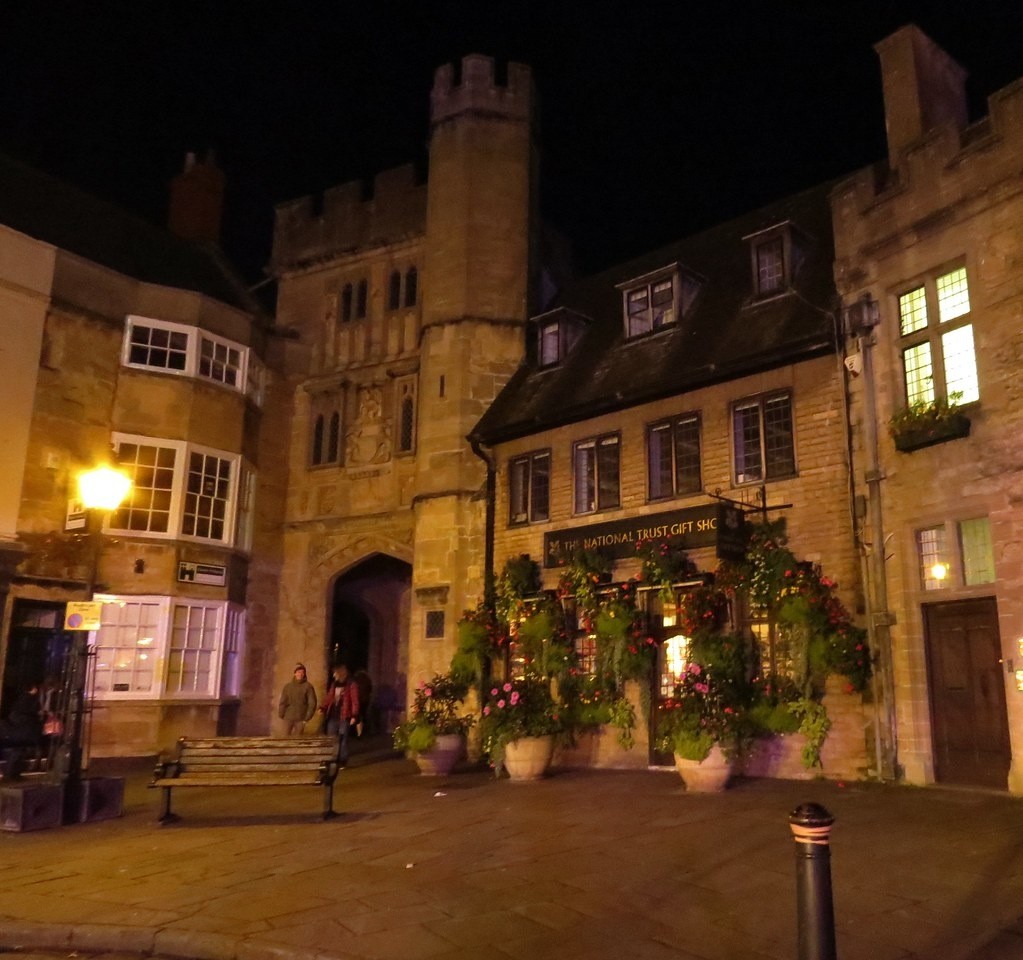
[339,762,346,771]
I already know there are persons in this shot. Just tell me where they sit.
[318,667,360,771]
[4,681,53,782]
[279,663,317,737]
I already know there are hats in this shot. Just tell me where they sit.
[293,662,306,674]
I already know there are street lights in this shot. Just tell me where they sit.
[56,463,132,826]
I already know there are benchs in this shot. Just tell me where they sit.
[147,735,341,823]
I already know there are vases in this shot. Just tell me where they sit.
[894,416,970,452]
[416,734,465,777]
[503,734,556,782]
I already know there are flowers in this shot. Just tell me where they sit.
[391,534,866,782]
[674,742,737,792]
[883,393,960,436]
